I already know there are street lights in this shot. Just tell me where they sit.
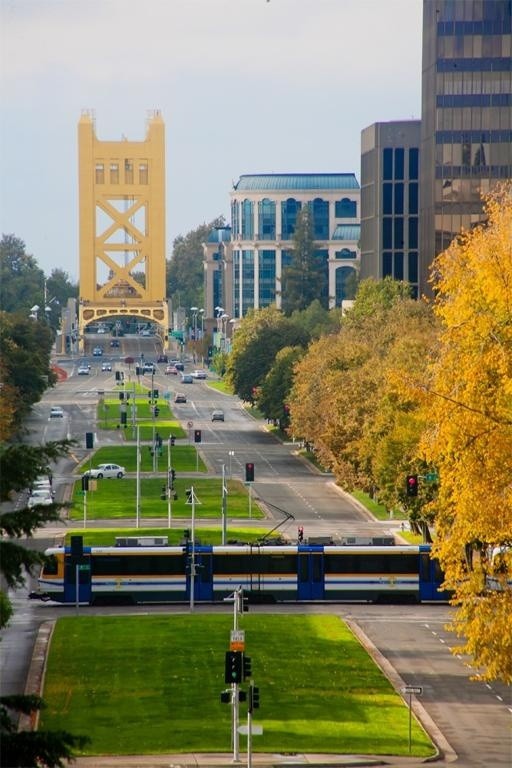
[213,457,228,544]
[27,304,51,328]
[189,306,237,368]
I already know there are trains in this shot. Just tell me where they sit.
[32,543,474,603]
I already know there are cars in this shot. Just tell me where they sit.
[96,322,154,336]
[212,409,225,422]
[109,339,119,346]
[101,360,112,371]
[92,343,104,356]
[50,405,64,417]
[85,461,126,480]
[175,392,186,402]
[27,465,56,514]
[78,358,91,375]
[144,354,207,384]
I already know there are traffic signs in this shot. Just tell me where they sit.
[399,685,425,696]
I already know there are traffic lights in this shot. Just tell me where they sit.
[237,590,250,614]
[171,469,176,481]
[184,485,193,504]
[154,389,158,397]
[225,649,241,684]
[155,407,160,416]
[249,685,259,708]
[180,527,205,576]
[194,430,201,442]
[242,651,252,681]
[171,435,177,445]
[297,526,304,541]
[245,463,254,481]
[406,474,418,496]
